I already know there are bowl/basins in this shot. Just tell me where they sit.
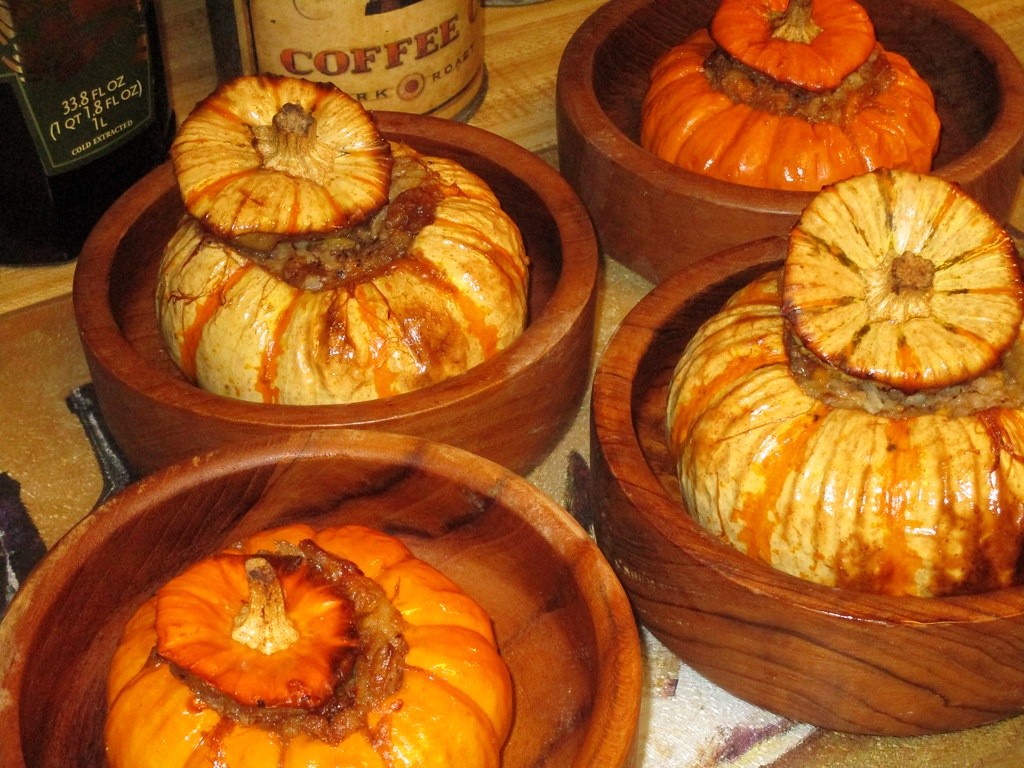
[555,0,1024,287]
[71,109,599,479]
[0,427,644,768]
[588,233,1024,738]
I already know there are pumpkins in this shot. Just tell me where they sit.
[101,0,1024,768]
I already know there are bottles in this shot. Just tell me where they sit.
[203,0,489,124]
[0,0,178,267]
